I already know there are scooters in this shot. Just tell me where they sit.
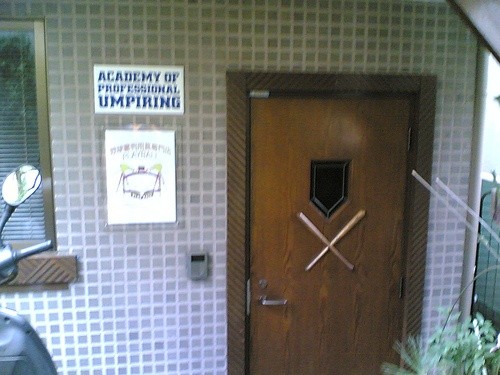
[0,163,63,375]
